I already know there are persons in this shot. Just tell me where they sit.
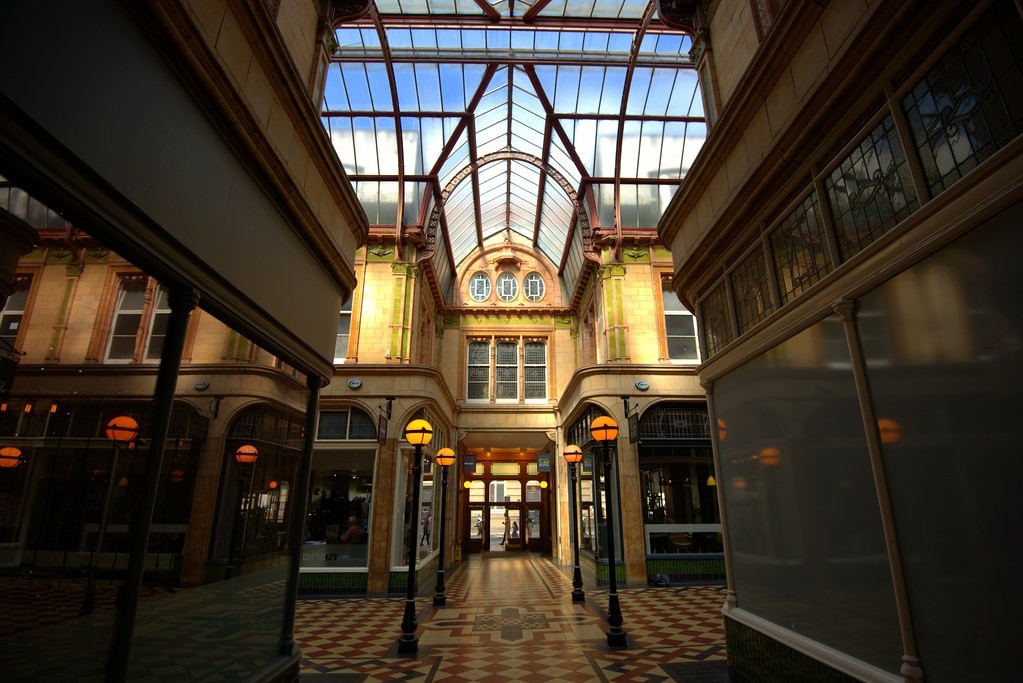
[499,513,511,545]
[582,516,587,535]
[512,521,519,538]
[340,516,364,544]
[420,512,431,546]
[477,512,482,536]
[526,518,533,538]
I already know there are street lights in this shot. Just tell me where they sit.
[396,419,434,652]
[759,449,789,585]
[75,415,141,622]
[563,445,585,602]
[433,447,457,606]
[590,416,628,648]
[219,445,260,608]
